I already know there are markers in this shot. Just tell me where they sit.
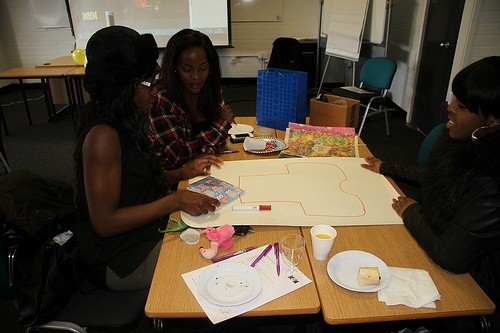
[222,150,239,153]
[232,205,272,211]
[221,101,237,124]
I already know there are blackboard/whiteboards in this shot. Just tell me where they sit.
[324,0,370,61]
[318,0,391,47]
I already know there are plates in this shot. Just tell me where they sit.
[197,262,263,306]
[326,250,391,293]
[247,135,289,154]
[228,124,254,135]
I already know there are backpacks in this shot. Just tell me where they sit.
[265,38,316,97]
[0,209,99,327]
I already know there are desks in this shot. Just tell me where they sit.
[145,117,496,333]
[0,55,90,141]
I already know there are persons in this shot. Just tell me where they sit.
[144,29,235,190]
[72,26,223,293]
[361,56,500,283]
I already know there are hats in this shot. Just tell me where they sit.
[84,26,160,101]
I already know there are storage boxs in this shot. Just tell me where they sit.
[310,95,360,127]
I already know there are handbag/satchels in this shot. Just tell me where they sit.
[255,68,307,131]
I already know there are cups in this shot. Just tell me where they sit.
[279,234,306,266]
[310,224,337,260]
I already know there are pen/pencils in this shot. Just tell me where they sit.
[274,241,280,276]
[251,243,272,267]
[211,247,254,262]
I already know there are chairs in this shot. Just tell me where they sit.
[332,57,397,136]
[37,211,147,333]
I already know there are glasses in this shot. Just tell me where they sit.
[139,72,163,89]
